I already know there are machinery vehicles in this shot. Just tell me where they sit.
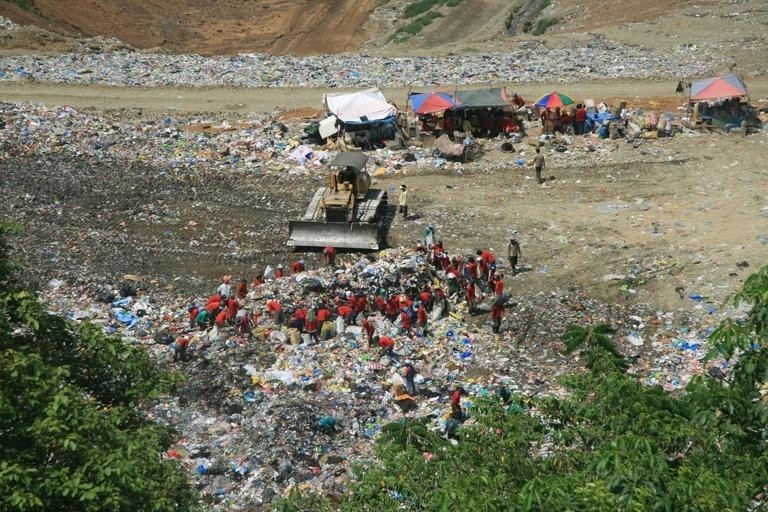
[285,151,388,251]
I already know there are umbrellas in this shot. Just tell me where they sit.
[534,91,574,109]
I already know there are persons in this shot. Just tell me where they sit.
[532,149,545,183]
[541,104,587,135]
[343,166,353,182]
[513,93,524,108]
[399,185,409,220]
[415,106,504,138]
[509,235,521,275]
[173,336,188,360]
